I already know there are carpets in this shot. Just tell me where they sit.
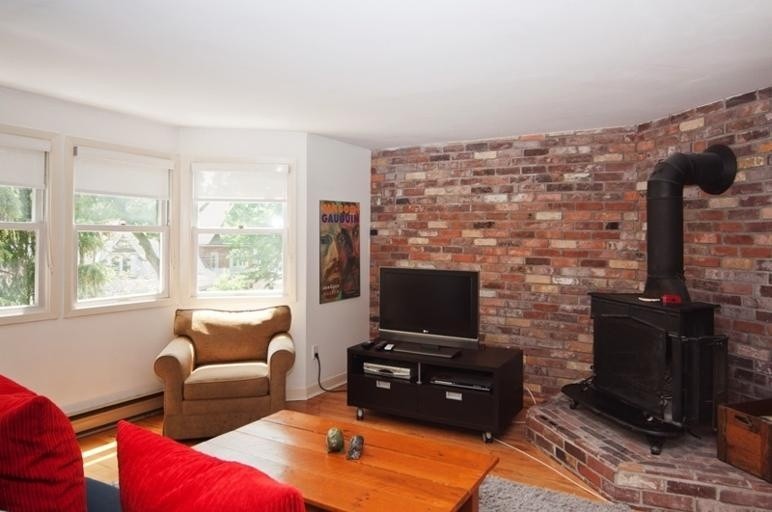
[478,471,640,512]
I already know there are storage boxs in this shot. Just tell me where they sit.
[717,398,772,484]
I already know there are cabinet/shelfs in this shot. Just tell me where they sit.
[346,341,524,443]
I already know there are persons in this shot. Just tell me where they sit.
[319,200,353,304]
[340,202,360,299]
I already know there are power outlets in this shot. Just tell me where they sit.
[312,345,320,360]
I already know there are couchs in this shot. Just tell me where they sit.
[83,473,122,511]
[154,304,295,440]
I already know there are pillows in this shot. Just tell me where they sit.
[0,372,89,512]
[115,420,306,512]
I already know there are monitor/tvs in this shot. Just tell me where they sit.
[378,266,480,358]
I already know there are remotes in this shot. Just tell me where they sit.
[375,341,388,350]
[384,343,395,350]
